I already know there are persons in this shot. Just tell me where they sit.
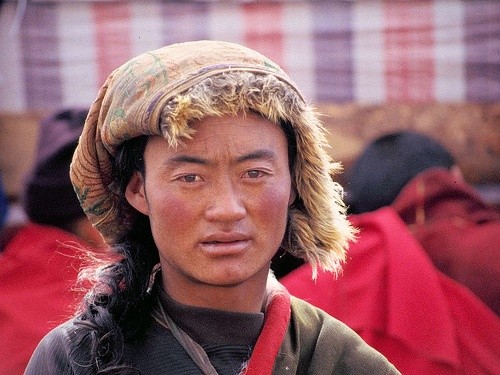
[270,129,500,375]
[23,40,403,375]
[0,106,120,375]
